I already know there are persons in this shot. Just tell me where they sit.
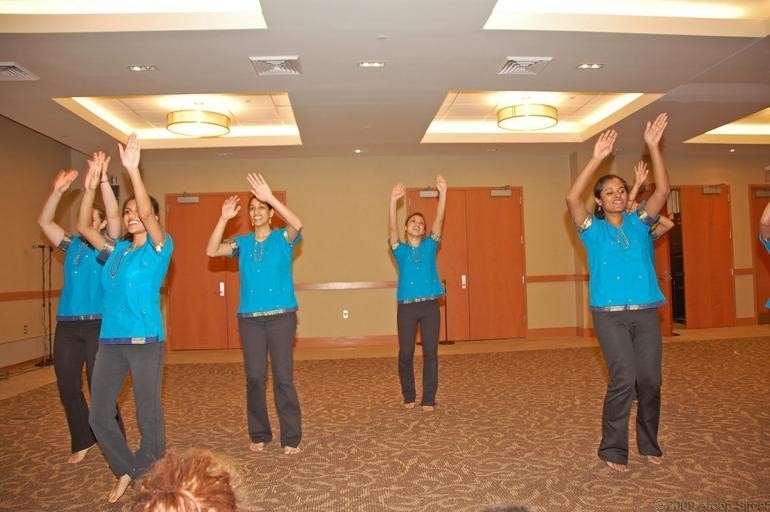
[205,170,302,457]
[755,200,770,310]
[565,110,675,479]
[624,152,676,405]
[38,149,129,466]
[74,132,175,503]
[387,174,448,414]
[122,447,252,511]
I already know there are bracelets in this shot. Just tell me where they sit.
[99,179,109,184]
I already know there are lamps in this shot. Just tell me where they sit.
[165,109,231,139]
[496,104,559,132]
